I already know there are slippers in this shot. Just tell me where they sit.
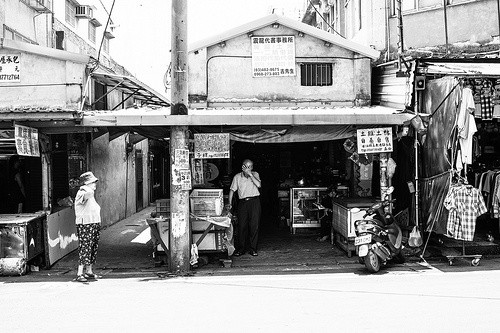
[248,250,258,256]
[234,251,245,256]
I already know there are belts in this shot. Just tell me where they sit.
[242,197,257,201]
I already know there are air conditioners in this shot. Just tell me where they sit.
[76,6,91,18]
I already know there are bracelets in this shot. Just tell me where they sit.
[249,173,253,177]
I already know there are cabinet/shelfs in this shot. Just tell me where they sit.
[279,187,327,233]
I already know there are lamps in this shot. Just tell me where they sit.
[324,41,331,47]
[272,23,280,29]
[298,32,304,37]
[219,42,225,47]
[246,32,253,38]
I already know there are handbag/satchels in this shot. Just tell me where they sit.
[408,226,423,247]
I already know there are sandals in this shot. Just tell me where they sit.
[76,274,87,281]
[85,273,98,278]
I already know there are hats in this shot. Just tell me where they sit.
[79,171,98,185]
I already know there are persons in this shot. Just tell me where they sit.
[74,172,102,281]
[225,159,262,256]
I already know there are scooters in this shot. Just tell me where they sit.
[353,186,406,273]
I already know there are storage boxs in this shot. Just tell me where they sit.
[189,189,224,216]
[156,199,170,217]
